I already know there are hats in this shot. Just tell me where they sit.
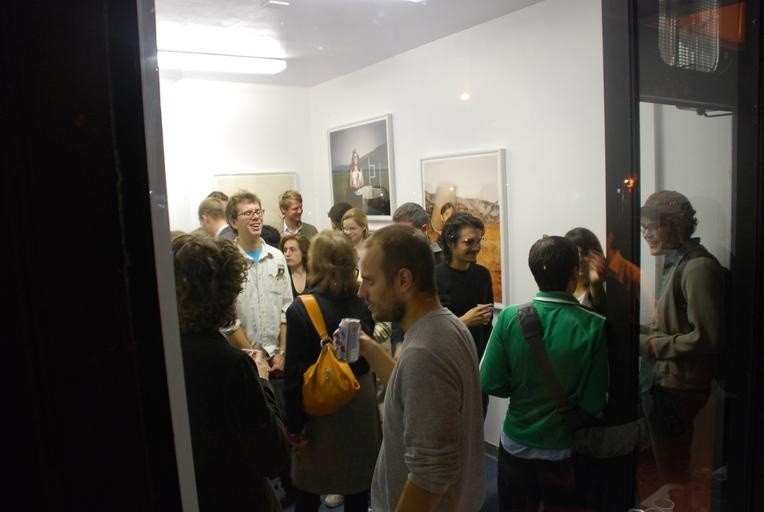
[641,188,696,228]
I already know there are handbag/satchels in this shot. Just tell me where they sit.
[297,294,360,419]
[518,306,600,477]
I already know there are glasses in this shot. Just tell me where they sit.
[641,223,660,233]
[237,209,265,217]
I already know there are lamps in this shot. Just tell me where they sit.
[157,51,286,74]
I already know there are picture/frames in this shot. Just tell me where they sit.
[420,149,507,310]
[327,113,395,221]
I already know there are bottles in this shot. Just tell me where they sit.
[335,317,361,364]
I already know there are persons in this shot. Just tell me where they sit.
[218,192,295,416]
[198,198,238,242]
[348,150,382,214]
[275,189,318,243]
[339,207,371,281]
[190,191,229,236]
[564,226,609,313]
[638,189,721,444]
[332,223,488,512]
[434,211,495,422]
[171,233,292,512]
[283,229,383,512]
[281,234,313,300]
[479,235,612,512]
[430,201,455,244]
[389,202,442,360]
